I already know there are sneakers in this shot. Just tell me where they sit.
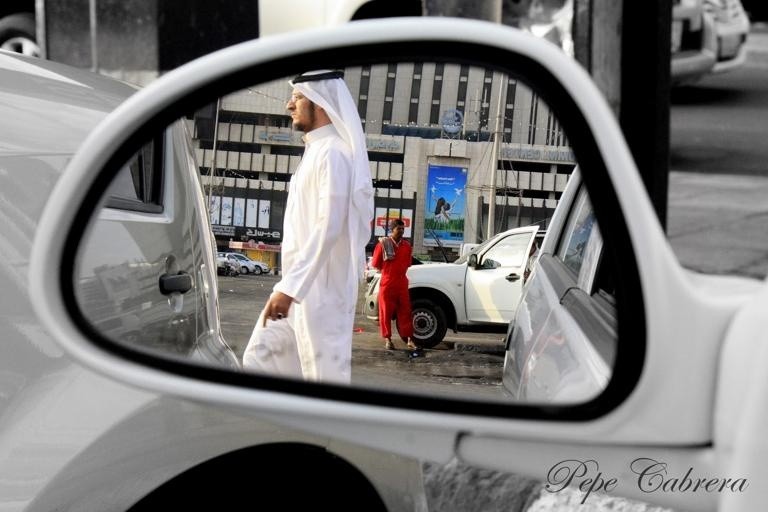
[385,341,395,350]
[407,342,423,350]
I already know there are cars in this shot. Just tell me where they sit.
[0,48,426,512]
[75,118,240,371]
[30,0,768,512]
[364,225,547,346]
[217,252,270,277]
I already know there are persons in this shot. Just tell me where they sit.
[236,68,377,392]
[369,219,436,345]
[434,197,450,223]
[433,196,457,222]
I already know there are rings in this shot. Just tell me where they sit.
[276,312,284,319]
